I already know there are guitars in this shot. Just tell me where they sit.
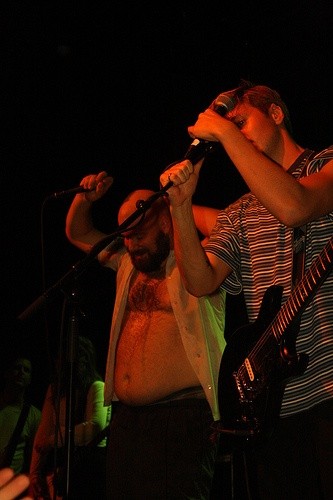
[217,234,333,429]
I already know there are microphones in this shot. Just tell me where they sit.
[183,92,237,161]
[54,176,113,197]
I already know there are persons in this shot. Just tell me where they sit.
[0,333,112,500]
[65,171,228,500]
[160,86,333,500]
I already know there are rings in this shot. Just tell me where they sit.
[168,172,172,181]
